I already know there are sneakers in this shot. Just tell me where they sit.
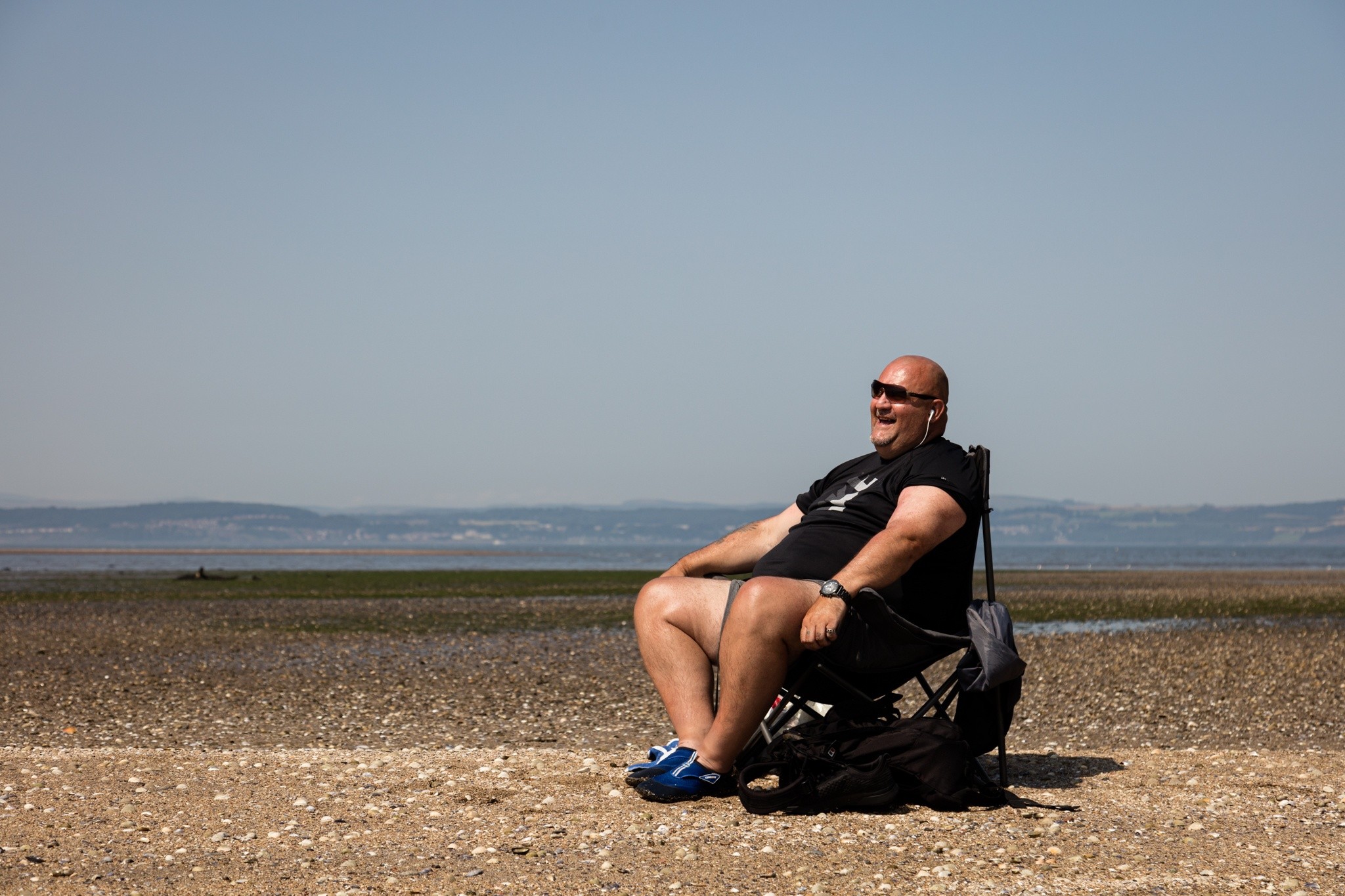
[634,751,736,804]
[625,746,696,787]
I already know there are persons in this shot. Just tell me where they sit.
[624,355,979,803]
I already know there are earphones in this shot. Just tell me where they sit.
[929,409,935,421]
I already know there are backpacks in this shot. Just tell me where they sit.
[737,711,957,816]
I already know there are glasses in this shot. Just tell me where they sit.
[871,379,939,401]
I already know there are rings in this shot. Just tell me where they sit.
[826,629,836,634]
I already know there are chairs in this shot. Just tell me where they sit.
[711,445,1008,805]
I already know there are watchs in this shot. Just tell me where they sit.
[820,579,852,607]
